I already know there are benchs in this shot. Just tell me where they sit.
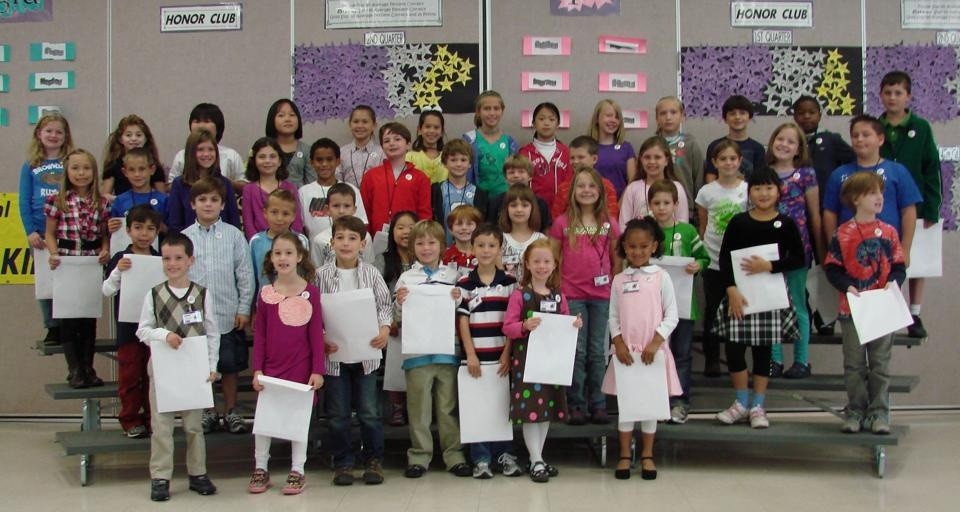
[33,328,928,486]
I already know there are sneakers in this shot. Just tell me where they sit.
[124,425,147,438]
[448,453,557,482]
[667,405,688,424]
[189,475,216,495]
[405,464,425,478]
[841,409,889,434]
[200,408,247,434]
[390,401,405,426]
[333,458,383,485]
[567,410,608,424]
[769,361,810,378]
[282,471,305,494]
[715,399,769,428]
[248,469,269,493]
[151,479,169,500]
[907,315,926,338]
[44,326,61,345]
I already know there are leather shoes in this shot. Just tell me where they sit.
[67,368,104,388]
[615,458,656,479]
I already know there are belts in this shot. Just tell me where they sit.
[58,238,100,249]
